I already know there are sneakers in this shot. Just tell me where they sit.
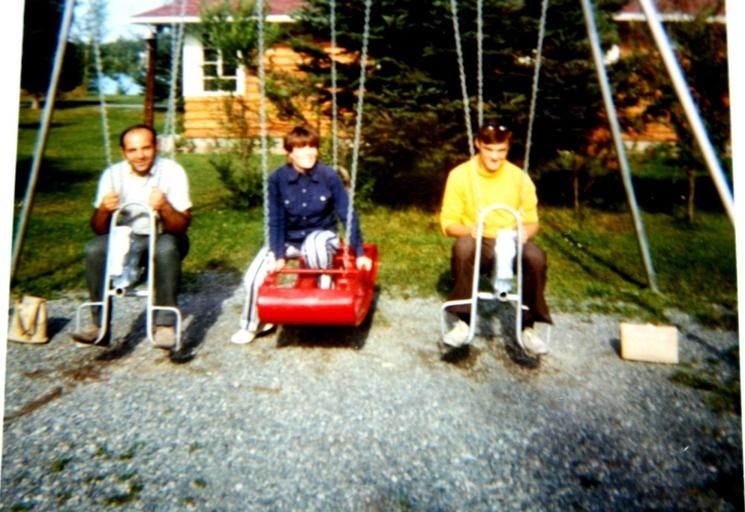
[69,329,111,346]
[443,319,469,348]
[523,327,549,355]
[153,325,176,347]
[232,322,274,344]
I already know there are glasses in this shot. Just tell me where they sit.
[482,124,508,131]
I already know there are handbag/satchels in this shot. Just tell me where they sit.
[8,295,49,344]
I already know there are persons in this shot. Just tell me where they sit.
[438,121,556,355]
[230,127,373,345]
[67,124,194,349]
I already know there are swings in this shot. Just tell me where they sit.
[446,0,550,361]
[256,1,378,325]
[71,0,182,356]
